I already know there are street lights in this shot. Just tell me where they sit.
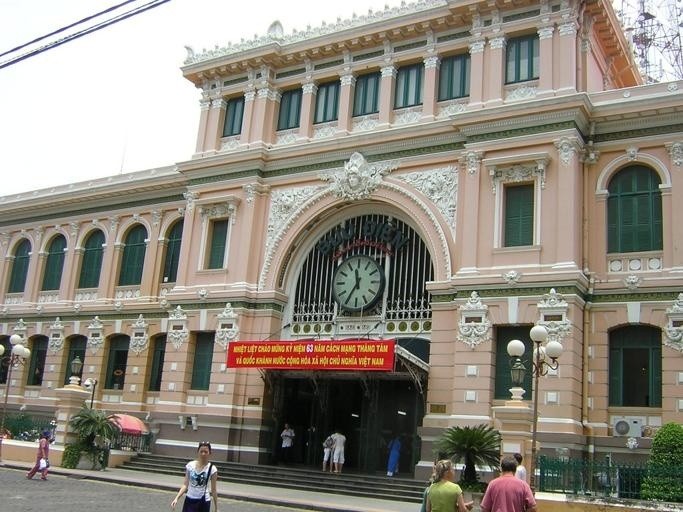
[506,321,562,499]
[84,378,96,410]
[0,334,31,459]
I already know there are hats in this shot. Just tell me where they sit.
[42,430,52,437]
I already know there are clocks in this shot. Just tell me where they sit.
[331,255,385,313]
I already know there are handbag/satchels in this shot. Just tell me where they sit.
[38,457,49,471]
[193,495,210,512]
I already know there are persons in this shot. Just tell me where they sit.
[479,455,537,511]
[329,429,346,472]
[512,452,526,483]
[322,436,334,472]
[169,441,218,512]
[279,423,294,466]
[425,459,474,512]
[386,432,400,476]
[420,466,436,511]
[25,430,51,480]
